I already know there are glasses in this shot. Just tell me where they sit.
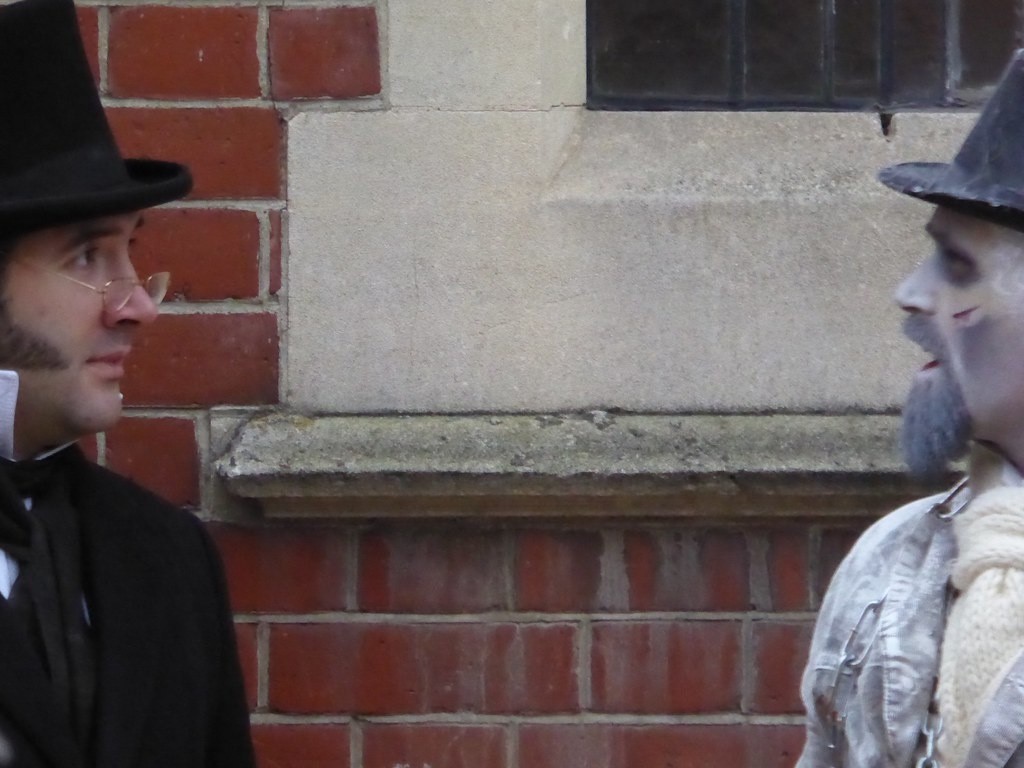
[11,255,171,313]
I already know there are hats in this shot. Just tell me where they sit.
[878,48,1024,211]
[0,0,193,232]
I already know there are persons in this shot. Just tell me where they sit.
[793,49,1024,768]
[0,0,258,768]
